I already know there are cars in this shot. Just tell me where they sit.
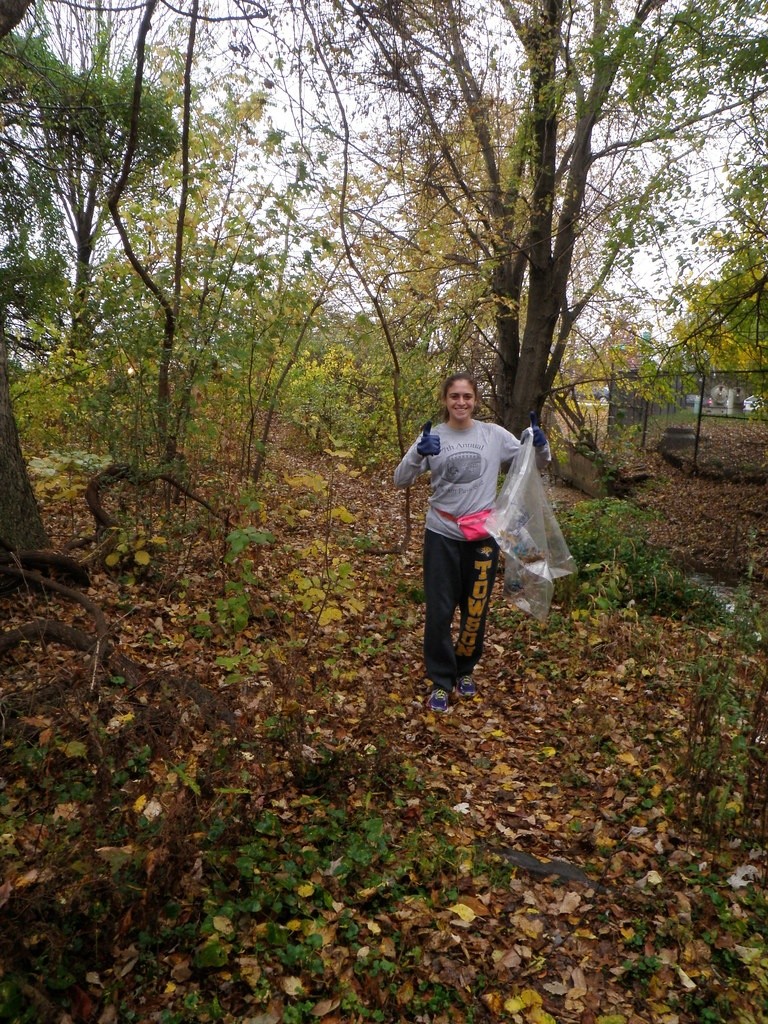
[725,394,765,414]
[573,386,611,403]
[686,395,713,408]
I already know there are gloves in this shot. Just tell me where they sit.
[417,421,440,456]
[525,426,547,447]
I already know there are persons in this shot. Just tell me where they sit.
[394,373,549,712]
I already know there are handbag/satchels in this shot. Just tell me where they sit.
[433,508,498,541]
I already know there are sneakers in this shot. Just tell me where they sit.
[430,688,449,713]
[456,675,477,698]
